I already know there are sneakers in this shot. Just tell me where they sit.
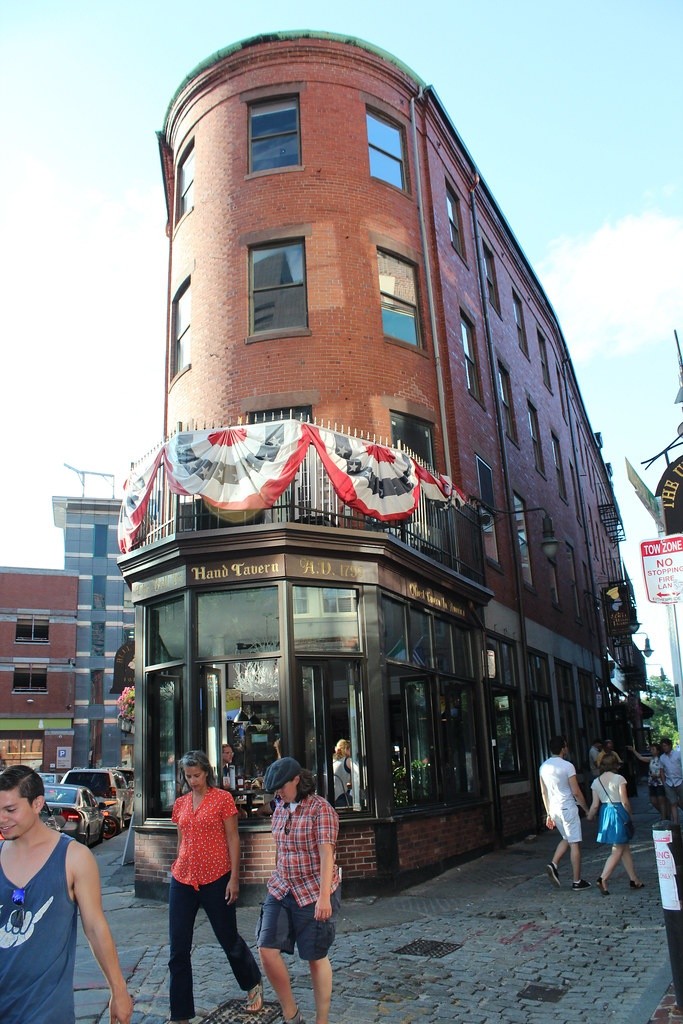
[546,863,560,888]
[572,879,592,890]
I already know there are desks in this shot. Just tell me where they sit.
[231,788,275,820]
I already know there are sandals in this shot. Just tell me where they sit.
[246,979,264,1014]
[282,1009,305,1024]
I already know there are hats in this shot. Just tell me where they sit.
[264,757,301,791]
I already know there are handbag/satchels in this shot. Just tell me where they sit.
[624,819,635,840]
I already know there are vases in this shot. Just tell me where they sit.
[392,786,409,808]
[411,766,431,803]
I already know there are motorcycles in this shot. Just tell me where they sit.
[97,801,120,840]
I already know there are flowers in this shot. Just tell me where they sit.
[409,757,432,767]
[118,686,135,723]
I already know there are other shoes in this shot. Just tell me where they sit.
[630,881,645,890]
[596,876,609,895]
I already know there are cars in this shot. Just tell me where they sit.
[43,783,106,847]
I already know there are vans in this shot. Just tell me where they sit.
[37,772,63,802]
[61,767,134,833]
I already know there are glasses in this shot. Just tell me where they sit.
[11,886,26,928]
[222,752,234,757]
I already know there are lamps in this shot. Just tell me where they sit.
[583,590,640,632]
[471,494,563,558]
[637,638,653,657]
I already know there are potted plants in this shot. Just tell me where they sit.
[394,768,408,781]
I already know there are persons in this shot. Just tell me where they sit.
[660,739,683,823]
[252,738,284,820]
[587,751,645,895]
[603,740,622,772]
[589,740,603,780]
[0,765,134,1024]
[169,750,263,1024]
[626,743,670,820]
[540,736,594,891]
[222,744,247,819]
[256,757,341,1024]
[333,739,353,807]
